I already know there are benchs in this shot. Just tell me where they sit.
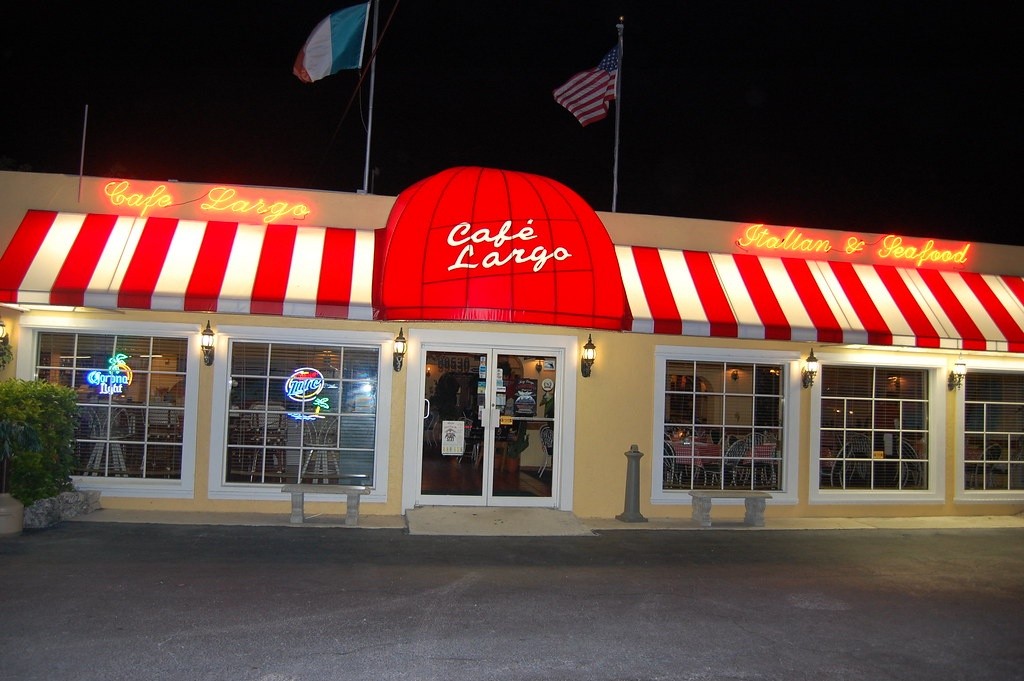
[281,485,373,526]
[688,490,773,527]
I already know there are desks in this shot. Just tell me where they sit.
[741,443,833,488]
[674,440,724,487]
[913,441,1012,487]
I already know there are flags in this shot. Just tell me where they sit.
[552,34,624,126]
[293,1,371,82]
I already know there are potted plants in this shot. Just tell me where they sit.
[507,440,528,472]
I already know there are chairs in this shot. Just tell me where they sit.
[456,416,482,464]
[427,395,448,447]
[543,360,555,371]
[662,418,1024,489]
[536,425,555,478]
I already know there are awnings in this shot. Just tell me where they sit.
[614,244,1024,353]
[0,209,385,322]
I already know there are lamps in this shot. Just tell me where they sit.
[804,347,819,387]
[0,321,12,348]
[391,326,407,372]
[583,333,599,378]
[201,320,217,366]
[426,366,432,377]
[730,369,740,379]
[535,360,543,374]
[949,352,967,389]
